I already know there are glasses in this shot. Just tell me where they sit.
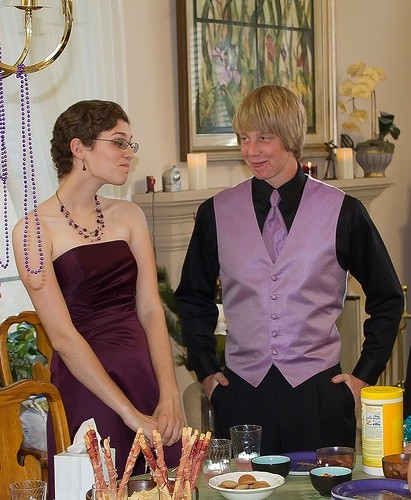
[93,138,139,154]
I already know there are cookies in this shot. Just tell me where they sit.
[218,475,270,490]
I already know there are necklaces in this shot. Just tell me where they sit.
[56,189,105,242]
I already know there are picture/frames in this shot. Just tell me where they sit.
[174,0,341,163]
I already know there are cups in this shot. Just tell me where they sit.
[203,439,232,490]
[230,424,262,472]
[9,480,47,500]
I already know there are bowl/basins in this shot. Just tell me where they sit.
[208,470,285,500]
[86,479,200,500]
[251,455,291,478]
[332,478,411,500]
[310,466,352,496]
[316,447,357,470]
[382,453,411,480]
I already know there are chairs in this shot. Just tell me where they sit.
[0,379,71,500]
[0,311,53,388]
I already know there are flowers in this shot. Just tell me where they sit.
[337,59,388,140]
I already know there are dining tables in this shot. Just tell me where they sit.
[115,459,385,500]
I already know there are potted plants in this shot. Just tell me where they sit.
[155,267,227,439]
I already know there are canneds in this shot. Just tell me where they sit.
[360,385,404,476]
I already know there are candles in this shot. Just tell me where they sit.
[303,160,317,180]
[334,147,353,179]
[187,153,207,190]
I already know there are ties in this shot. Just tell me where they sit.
[261,189,288,265]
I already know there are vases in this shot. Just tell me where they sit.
[355,138,394,177]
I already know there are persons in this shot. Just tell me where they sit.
[172,86,405,461]
[12,100,185,500]
